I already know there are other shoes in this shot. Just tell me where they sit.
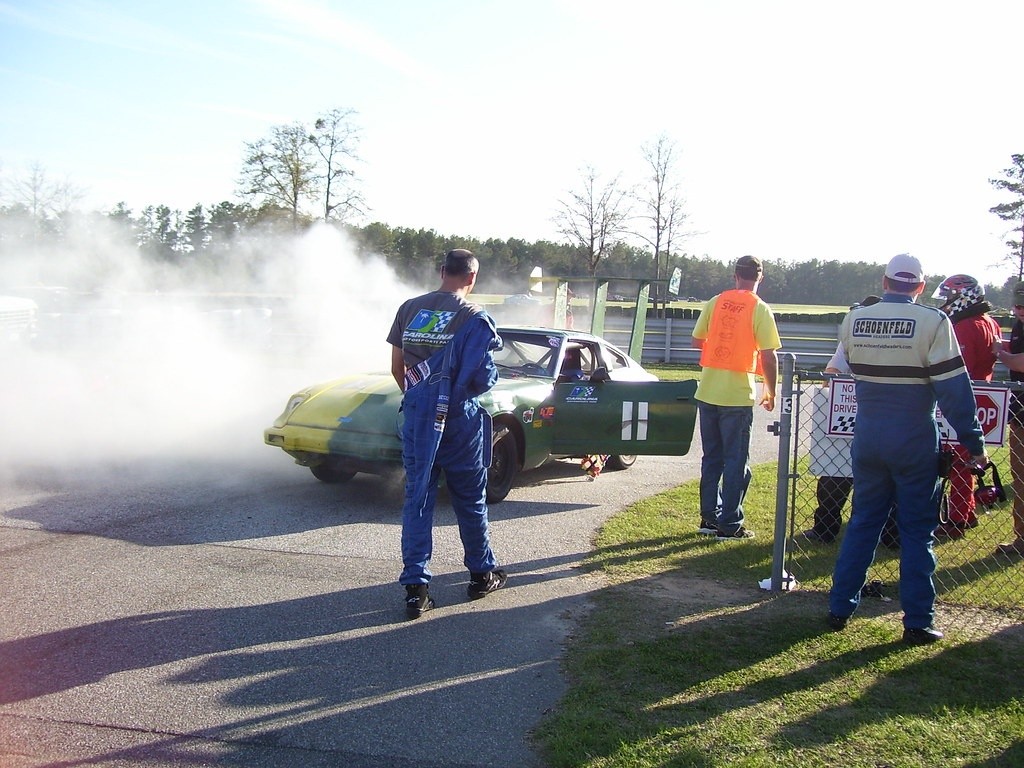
[714,528,755,540]
[825,606,850,631]
[996,536,1024,555]
[903,626,944,646]
[700,519,719,534]
[964,510,979,528]
[803,530,834,543]
[934,520,964,539]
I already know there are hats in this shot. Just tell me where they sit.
[885,253,925,283]
[1010,280,1024,306]
[733,255,763,280]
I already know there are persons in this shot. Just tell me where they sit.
[804,295,899,550]
[539,288,574,328]
[827,252,989,645]
[386,249,506,618]
[527,290,532,297]
[993,280,1024,555]
[690,256,782,540]
[930,275,1003,535]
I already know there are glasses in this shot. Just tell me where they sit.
[1013,304,1024,310]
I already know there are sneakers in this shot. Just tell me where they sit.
[467,570,507,600]
[404,584,435,619]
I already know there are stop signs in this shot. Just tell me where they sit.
[973,393,999,438]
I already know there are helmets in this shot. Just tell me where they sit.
[931,274,991,324]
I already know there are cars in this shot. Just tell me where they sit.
[504,294,541,306]
[263,325,697,503]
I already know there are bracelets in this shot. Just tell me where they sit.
[996,350,1004,357]
